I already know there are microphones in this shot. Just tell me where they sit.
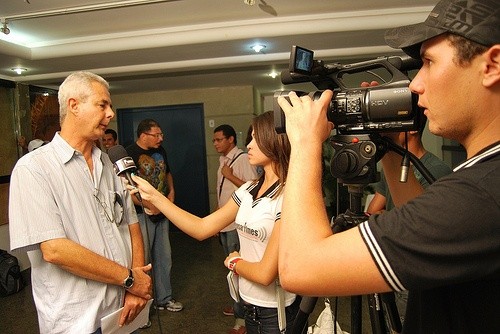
[281,62,351,84]
[107,145,142,202]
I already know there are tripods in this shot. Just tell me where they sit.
[291,132,404,334]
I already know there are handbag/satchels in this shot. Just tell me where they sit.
[0,249,25,297]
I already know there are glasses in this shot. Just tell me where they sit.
[141,132,163,138]
[211,136,227,144]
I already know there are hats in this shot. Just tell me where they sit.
[383,0,500,60]
[28,139,50,152]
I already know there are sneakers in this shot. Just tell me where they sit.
[140,319,152,329]
[232,326,247,334]
[223,306,235,315]
[152,297,183,311]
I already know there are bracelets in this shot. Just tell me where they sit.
[228,257,243,275]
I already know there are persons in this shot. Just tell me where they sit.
[368,116,452,327]
[278,0,500,334]
[103,129,118,153]
[19,137,47,152]
[8,72,152,334]
[213,124,258,334]
[125,119,183,329]
[124,111,308,334]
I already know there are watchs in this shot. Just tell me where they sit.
[123,269,134,289]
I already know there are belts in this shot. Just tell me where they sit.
[244,301,299,322]
